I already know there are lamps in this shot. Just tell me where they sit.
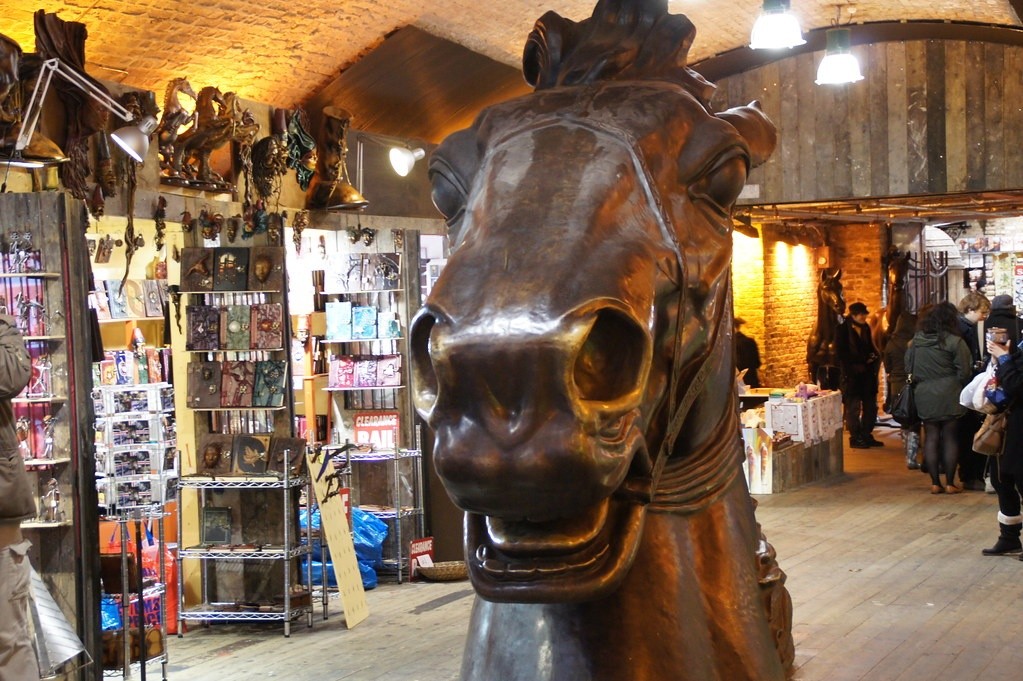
[351,132,428,210]
[0,56,161,170]
[777,220,799,247]
[792,222,814,246]
[814,6,867,89]
[748,0,807,52]
[733,216,761,240]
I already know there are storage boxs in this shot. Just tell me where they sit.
[763,386,843,449]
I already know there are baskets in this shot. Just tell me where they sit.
[415,560,468,581]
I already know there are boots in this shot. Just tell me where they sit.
[901,430,908,459]
[982,511,1023,555]
[906,432,921,469]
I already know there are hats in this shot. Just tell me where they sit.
[849,302,869,315]
[990,295,1013,308]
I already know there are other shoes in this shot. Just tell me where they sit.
[964,478,985,490]
[946,485,962,494]
[932,485,946,493]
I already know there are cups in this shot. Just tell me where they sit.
[985,327,1008,347]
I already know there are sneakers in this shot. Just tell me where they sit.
[985,476,996,492]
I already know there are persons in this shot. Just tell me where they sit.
[910,301,972,494]
[0,313,37,681]
[955,291,991,490]
[885,311,922,467]
[734,318,762,388]
[985,295,1023,356]
[837,302,884,448]
[982,338,1023,561]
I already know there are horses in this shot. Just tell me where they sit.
[806,269,847,388]
[404,0,796,680]
[869,250,918,415]
[150,74,262,184]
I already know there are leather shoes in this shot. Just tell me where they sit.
[865,435,883,447]
[849,437,869,448]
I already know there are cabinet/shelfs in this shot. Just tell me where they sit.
[316,289,416,448]
[99,496,182,681]
[171,221,294,473]
[167,447,316,638]
[0,245,72,535]
[320,422,427,586]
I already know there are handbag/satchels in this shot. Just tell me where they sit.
[108,519,187,633]
[972,412,1007,456]
[891,383,918,425]
[959,361,999,414]
